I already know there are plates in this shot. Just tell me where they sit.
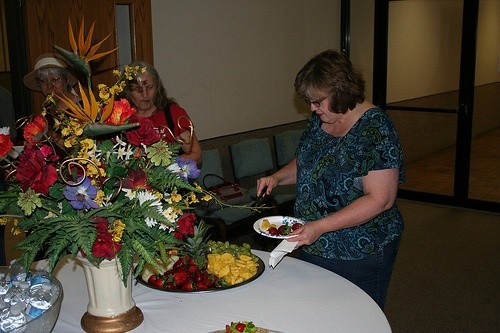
[133,253,266,295]
[253,216,306,239]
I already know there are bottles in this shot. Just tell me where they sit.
[2,259,53,321]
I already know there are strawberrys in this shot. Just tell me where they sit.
[148,256,218,291]
[268,223,301,235]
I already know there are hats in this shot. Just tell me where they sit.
[23,53,78,91]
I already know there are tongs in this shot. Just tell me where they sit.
[250,186,267,214]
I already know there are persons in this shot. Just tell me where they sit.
[0,86,17,140]
[23,53,84,133]
[123,62,201,167]
[257,49,407,312]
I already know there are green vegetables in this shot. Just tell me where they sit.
[230,321,258,333]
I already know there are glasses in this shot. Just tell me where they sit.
[37,77,64,86]
[304,95,329,107]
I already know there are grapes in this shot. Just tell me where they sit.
[207,240,258,262]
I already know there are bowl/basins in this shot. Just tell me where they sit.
[0,265,63,333]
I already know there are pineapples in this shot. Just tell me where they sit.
[207,253,259,284]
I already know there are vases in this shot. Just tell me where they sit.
[73,253,145,333]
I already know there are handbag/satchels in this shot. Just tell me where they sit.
[200,173,252,211]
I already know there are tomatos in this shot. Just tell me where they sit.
[235,321,246,332]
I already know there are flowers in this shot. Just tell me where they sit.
[0,18,271,286]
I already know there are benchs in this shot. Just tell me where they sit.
[192,120,309,250]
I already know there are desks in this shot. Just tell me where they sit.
[0,247,393,333]
